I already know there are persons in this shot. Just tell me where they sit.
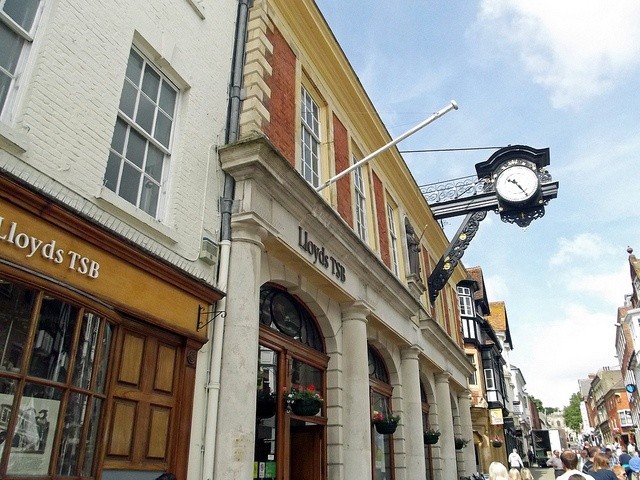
[508,448,525,472]
[527,449,533,467]
[546,431,640,480]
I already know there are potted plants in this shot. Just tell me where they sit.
[455,436,472,449]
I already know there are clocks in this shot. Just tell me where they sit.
[496,165,540,202]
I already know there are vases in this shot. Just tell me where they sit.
[375,424,397,434]
[424,436,438,444]
[289,400,320,416]
[492,443,502,447]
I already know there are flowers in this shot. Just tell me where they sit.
[490,435,504,443]
[423,427,441,436]
[371,406,402,423]
[282,383,324,415]
